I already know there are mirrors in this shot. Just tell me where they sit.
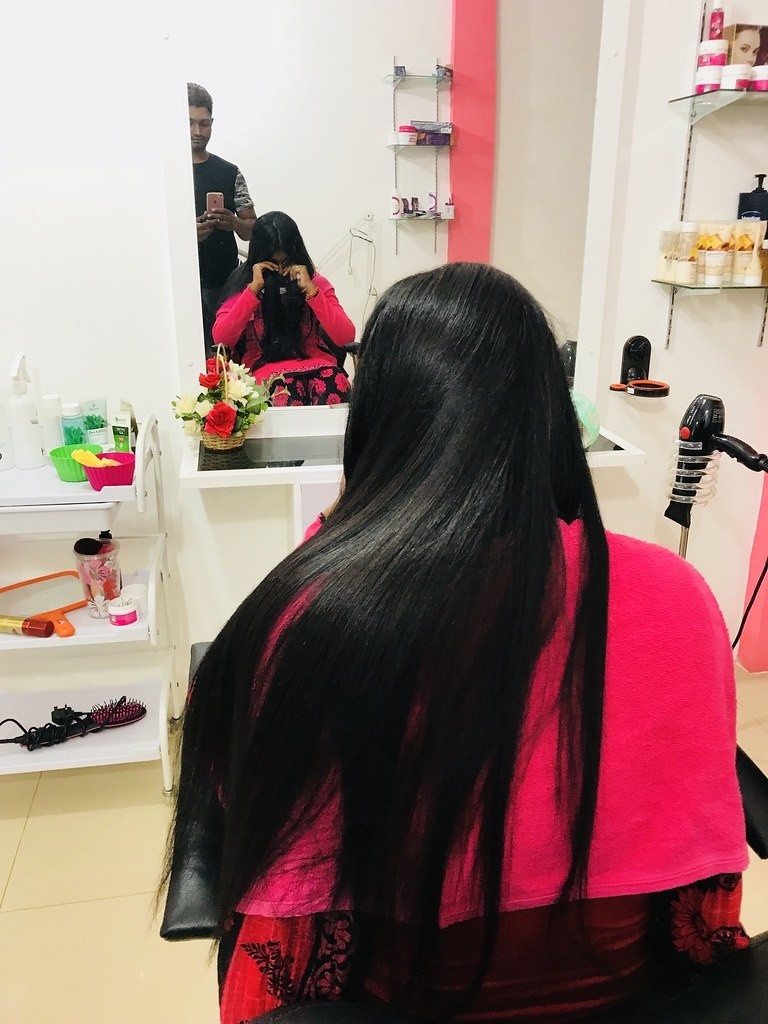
[182,0,605,412]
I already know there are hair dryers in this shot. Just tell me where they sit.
[664,394,768,529]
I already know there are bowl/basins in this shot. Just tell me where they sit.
[49,444,103,483]
[82,451,136,491]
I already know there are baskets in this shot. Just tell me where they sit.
[200,343,248,450]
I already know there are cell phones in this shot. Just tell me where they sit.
[207,192,224,217]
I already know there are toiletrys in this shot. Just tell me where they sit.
[0,352,141,472]
[390,187,400,220]
[656,174,768,289]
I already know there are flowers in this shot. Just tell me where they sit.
[206,353,250,374]
[170,369,291,440]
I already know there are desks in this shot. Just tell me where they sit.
[177,425,646,548]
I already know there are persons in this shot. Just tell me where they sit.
[732,24,768,67]
[187,80,357,404]
[155,260,750,1024]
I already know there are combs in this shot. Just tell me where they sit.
[25,698,146,748]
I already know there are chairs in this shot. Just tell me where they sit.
[158,640,768,1024]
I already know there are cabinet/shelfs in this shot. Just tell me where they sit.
[0,413,183,797]
[382,74,455,227]
[651,87,768,292]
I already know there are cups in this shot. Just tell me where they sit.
[73,538,122,618]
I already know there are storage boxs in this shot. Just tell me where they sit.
[722,23,768,67]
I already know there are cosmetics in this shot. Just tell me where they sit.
[0,615,55,637]
[656,175,768,286]
[40,395,139,453]
[390,188,436,218]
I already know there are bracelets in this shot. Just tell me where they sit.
[306,286,318,299]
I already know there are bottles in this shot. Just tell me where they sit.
[0,367,17,471]
[39,394,64,466]
[62,405,88,444]
[658,221,700,283]
[709,0,724,41]
